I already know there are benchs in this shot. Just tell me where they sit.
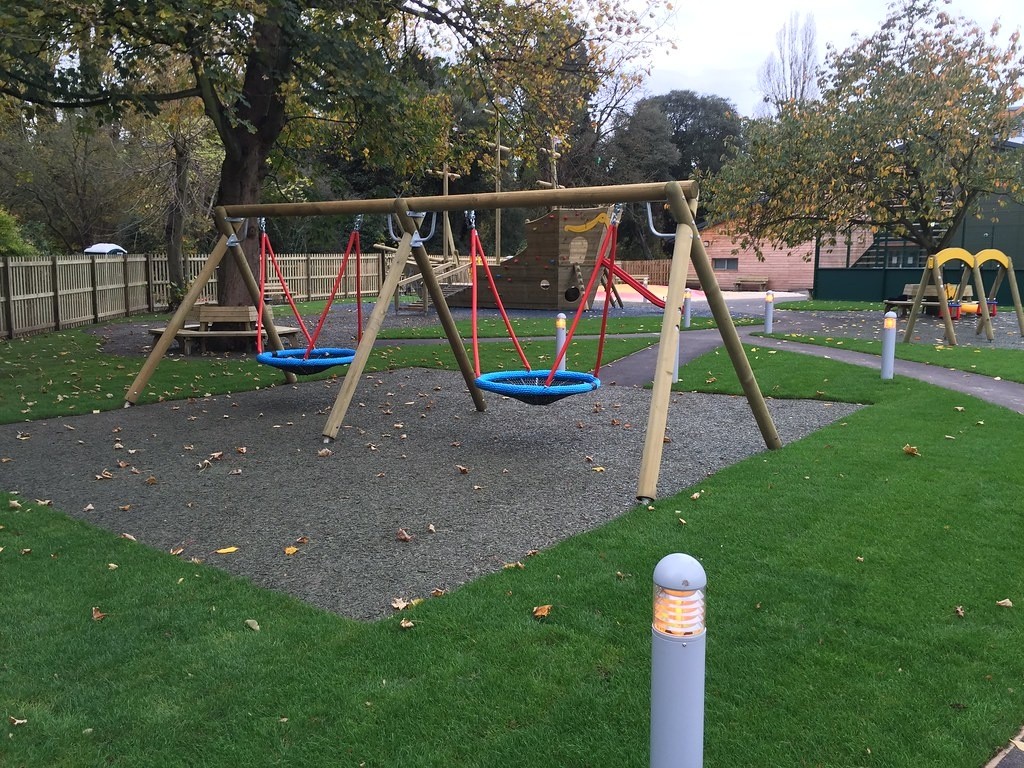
[883,284,980,318]
[263,283,295,303]
[732,275,769,291]
[147,305,301,355]
[686,274,702,290]
[614,274,650,284]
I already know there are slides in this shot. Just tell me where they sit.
[601,259,684,312]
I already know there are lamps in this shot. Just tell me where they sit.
[882,311,897,328]
[684,288,691,298]
[643,278,647,284]
[764,290,774,302]
[555,313,565,328]
[651,553,707,643]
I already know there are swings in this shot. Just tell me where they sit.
[938,263,964,319]
[976,265,999,317]
[464,203,626,406]
[255,214,362,375]
